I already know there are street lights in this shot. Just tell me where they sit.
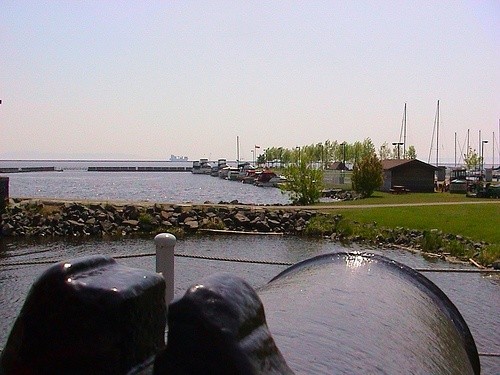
[319,144,323,171]
[340,143,345,166]
[481,140,488,171]
[296,146,300,166]
[251,145,260,167]
[392,142,404,159]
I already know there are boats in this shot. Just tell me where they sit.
[191,158,279,188]
